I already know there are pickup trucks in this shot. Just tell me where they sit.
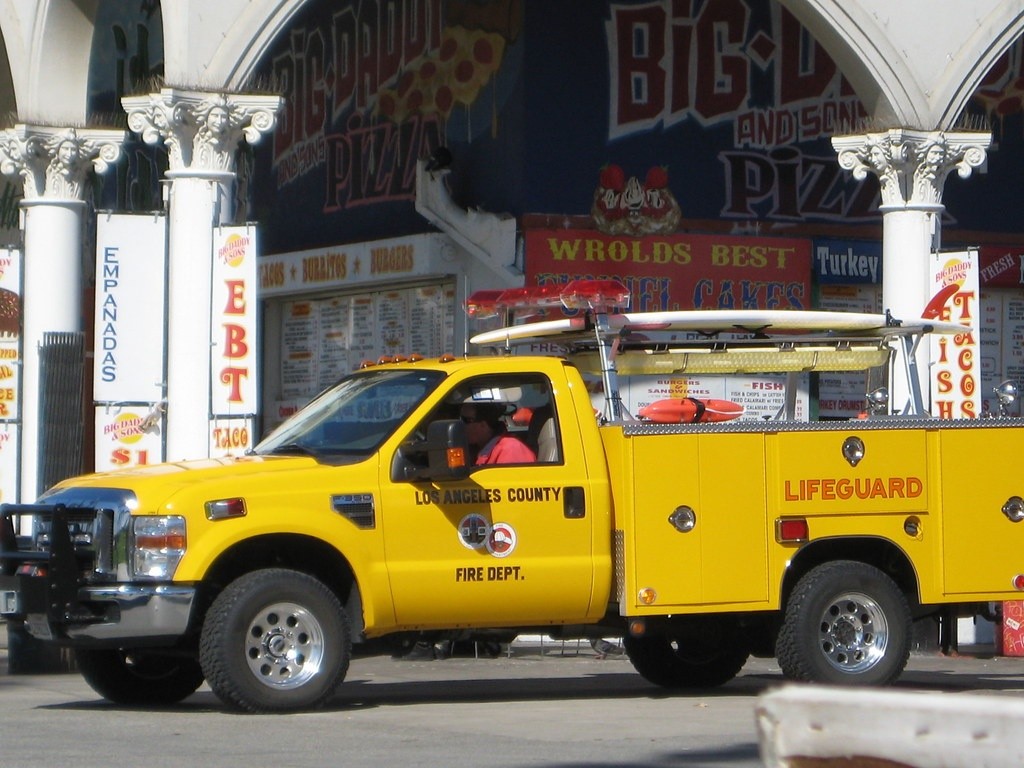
[1,281,1024,720]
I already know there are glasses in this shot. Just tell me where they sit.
[460,415,482,425]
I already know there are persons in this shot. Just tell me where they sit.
[148,102,183,168]
[7,134,36,198]
[43,130,95,200]
[191,96,245,170]
[911,131,956,204]
[459,402,538,464]
[858,137,892,204]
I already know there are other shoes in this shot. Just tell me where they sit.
[401,642,435,660]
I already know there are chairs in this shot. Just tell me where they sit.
[528,405,559,462]
